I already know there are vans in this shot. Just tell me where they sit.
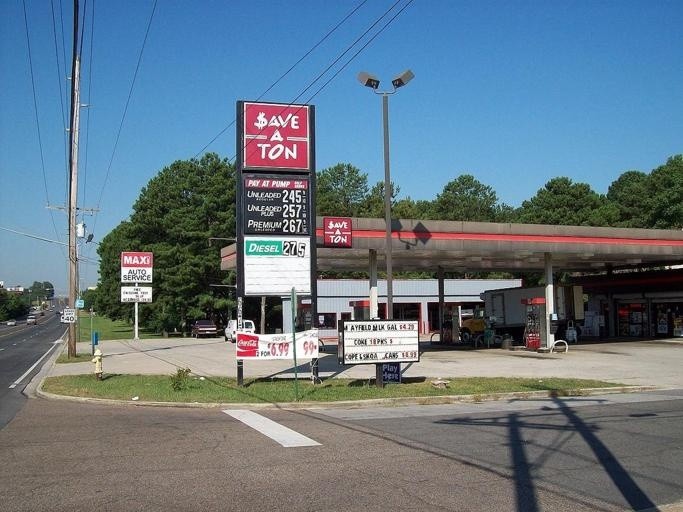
[27,316,36,325]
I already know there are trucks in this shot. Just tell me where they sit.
[461,284,586,343]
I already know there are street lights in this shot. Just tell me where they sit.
[356,71,416,388]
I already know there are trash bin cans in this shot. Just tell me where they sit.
[501,333,512,349]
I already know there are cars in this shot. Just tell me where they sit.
[26,304,38,312]
[49,304,60,314]
[60,315,64,323]
[191,319,255,343]
[40,312,44,316]
[7,319,16,326]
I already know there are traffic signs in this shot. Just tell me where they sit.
[64,309,75,324]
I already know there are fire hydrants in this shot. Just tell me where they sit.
[92,348,103,380]
